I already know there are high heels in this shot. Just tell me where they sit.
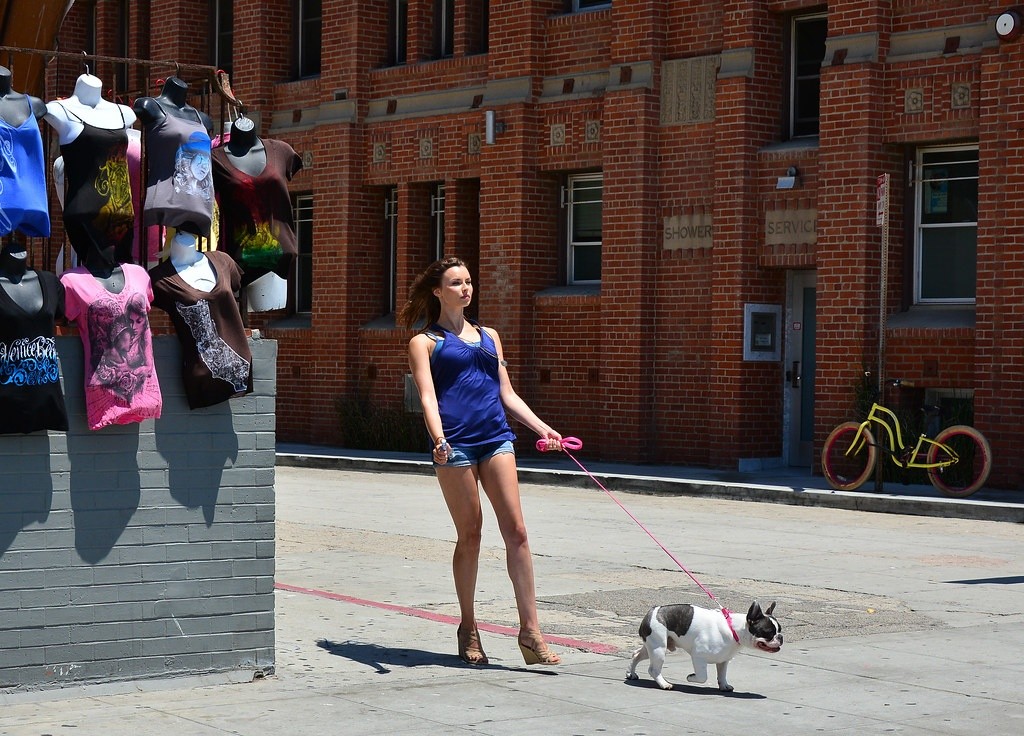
[457,623,488,665]
[518,628,561,665]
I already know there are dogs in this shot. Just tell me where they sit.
[626,600,784,692]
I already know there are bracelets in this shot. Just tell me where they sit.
[434,437,445,444]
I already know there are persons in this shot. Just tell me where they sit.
[398,257,565,669]
[213,116,307,265]
[0,66,51,240]
[211,121,234,147]
[148,233,253,411]
[153,198,220,260]
[59,263,162,431]
[126,128,166,271]
[53,156,82,278]
[133,75,215,237]
[0,243,69,435]
[42,73,138,279]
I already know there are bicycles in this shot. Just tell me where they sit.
[822,370,991,497]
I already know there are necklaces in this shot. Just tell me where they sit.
[458,327,507,367]
